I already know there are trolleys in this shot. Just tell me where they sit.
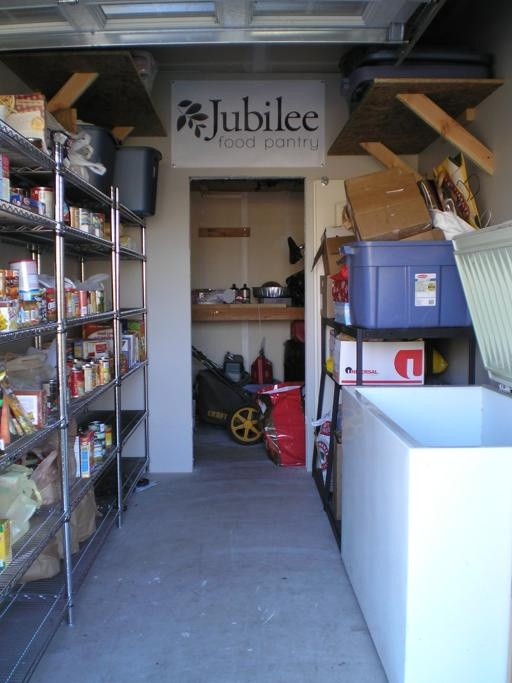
[190,347,264,444]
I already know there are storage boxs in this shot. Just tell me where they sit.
[316,167,472,331]
[114,144,163,217]
[332,334,427,388]
[331,430,342,521]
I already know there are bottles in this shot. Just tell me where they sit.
[239,283,250,303]
[230,283,239,303]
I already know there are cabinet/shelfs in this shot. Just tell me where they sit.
[311,317,475,551]
[1,119,151,683]
